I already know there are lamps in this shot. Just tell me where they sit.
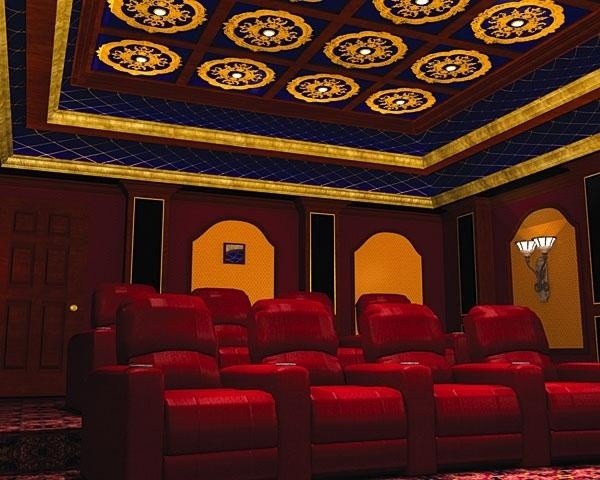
[514,236,557,303]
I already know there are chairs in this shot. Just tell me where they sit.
[68,284,600,480]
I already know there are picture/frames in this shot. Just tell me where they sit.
[223,243,245,265]
